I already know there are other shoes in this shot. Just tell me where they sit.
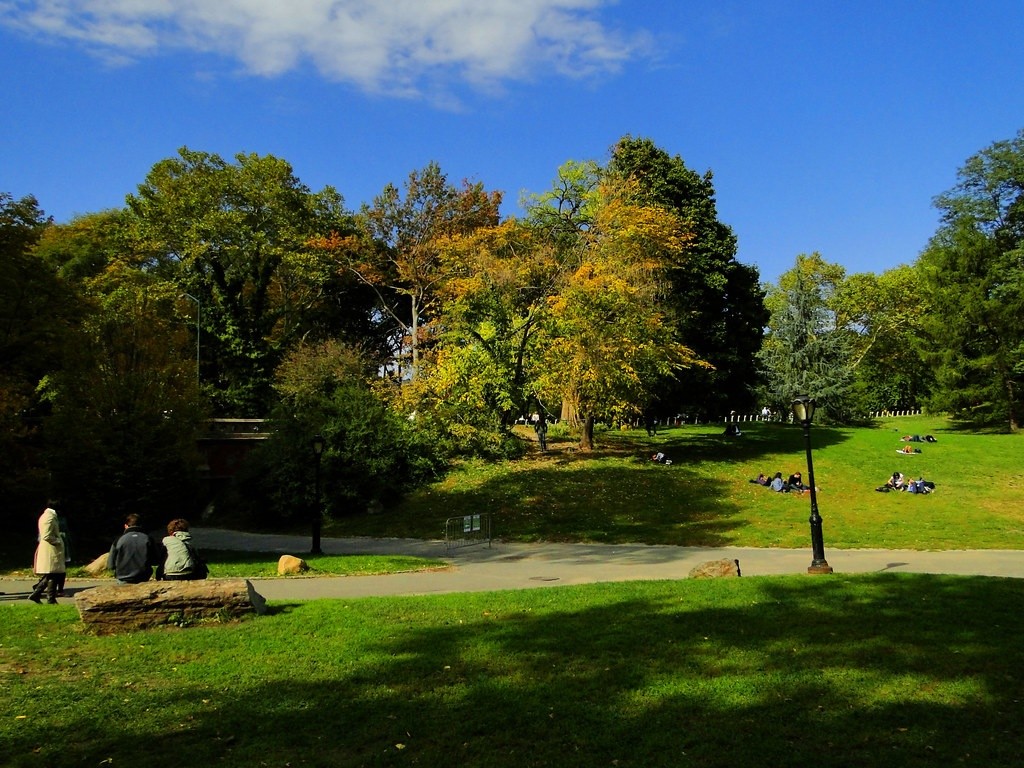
[57,590,72,597]
[47,597,57,604]
[27,593,43,605]
[32,583,39,591]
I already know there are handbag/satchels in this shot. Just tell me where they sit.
[187,546,209,580]
[106,543,117,570]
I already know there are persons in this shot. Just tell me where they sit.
[523,412,548,453]
[885,434,936,495]
[107,513,154,585]
[155,519,198,581]
[28,496,72,604]
[646,414,744,464]
[756,472,809,494]
